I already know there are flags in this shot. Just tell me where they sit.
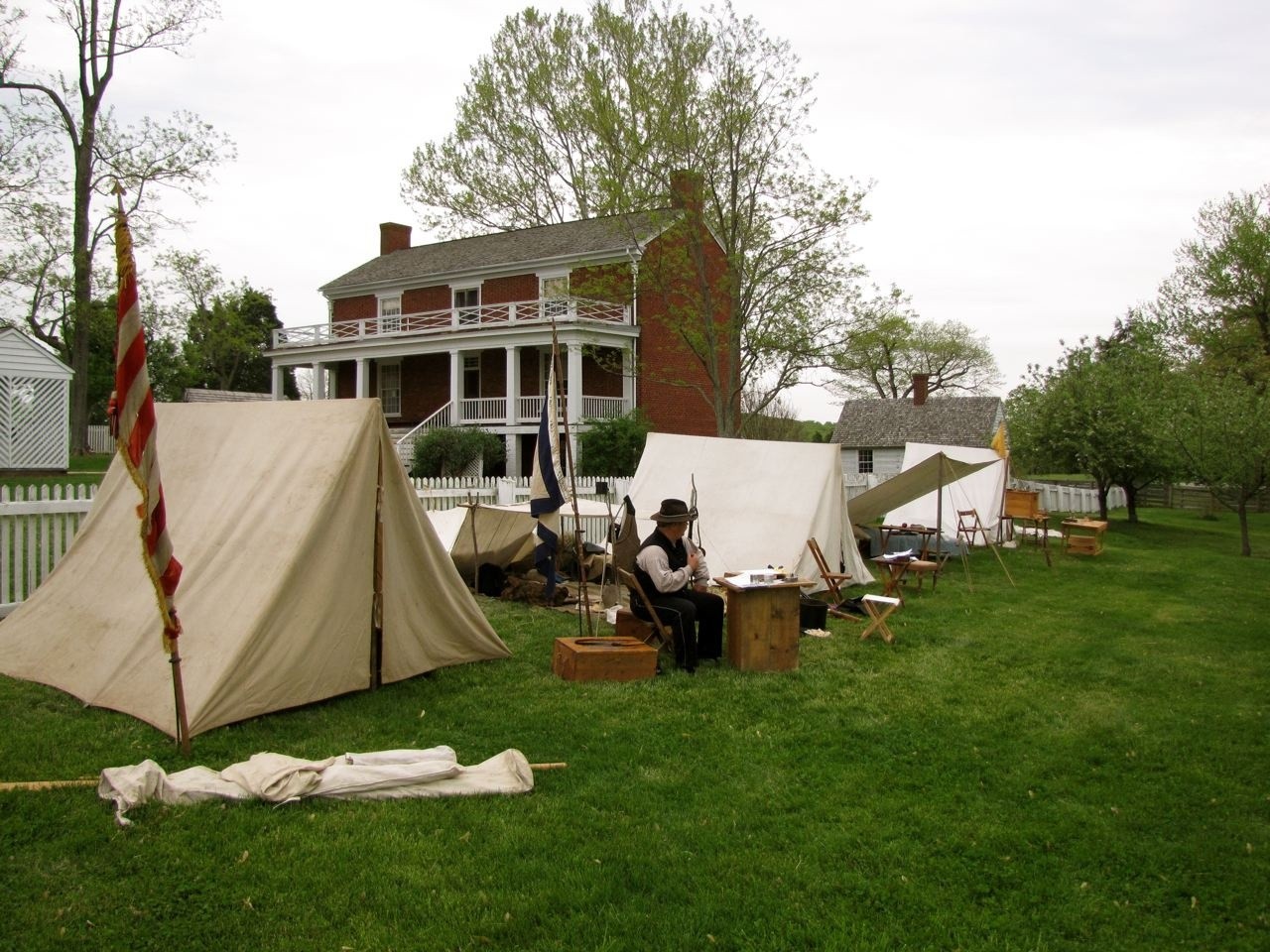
[109,212,184,654]
[531,353,572,599]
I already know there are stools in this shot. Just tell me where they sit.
[861,594,902,643]
[880,559,914,607]
[918,548,953,573]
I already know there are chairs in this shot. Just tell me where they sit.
[617,567,675,653]
[808,537,853,602]
[1022,508,1049,543]
[957,509,991,550]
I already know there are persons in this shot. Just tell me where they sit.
[626,499,724,673]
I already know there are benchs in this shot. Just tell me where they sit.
[873,558,938,592]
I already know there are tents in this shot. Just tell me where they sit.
[882,442,1016,546]
[596,432,876,598]
[0,397,512,744]
[426,497,624,603]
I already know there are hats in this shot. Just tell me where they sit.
[650,499,698,522]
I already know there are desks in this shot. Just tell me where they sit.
[997,514,1050,551]
[1062,518,1108,557]
[877,524,937,563]
[714,573,817,671]
